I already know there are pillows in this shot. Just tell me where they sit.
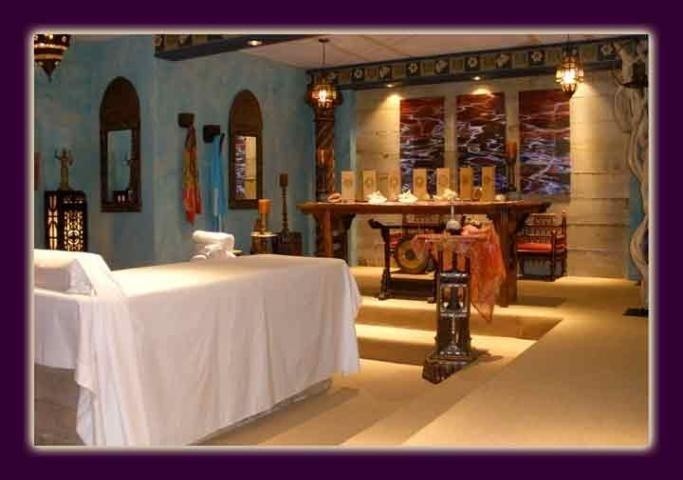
[34,249,106,294]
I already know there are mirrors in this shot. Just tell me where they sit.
[228,88,263,209]
[99,76,141,214]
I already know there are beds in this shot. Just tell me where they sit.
[31,254,361,446]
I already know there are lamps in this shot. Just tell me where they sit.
[555,36,584,97]
[34,34,70,79]
[307,35,340,118]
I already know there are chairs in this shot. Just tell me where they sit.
[368,218,449,302]
[516,211,569,282]
